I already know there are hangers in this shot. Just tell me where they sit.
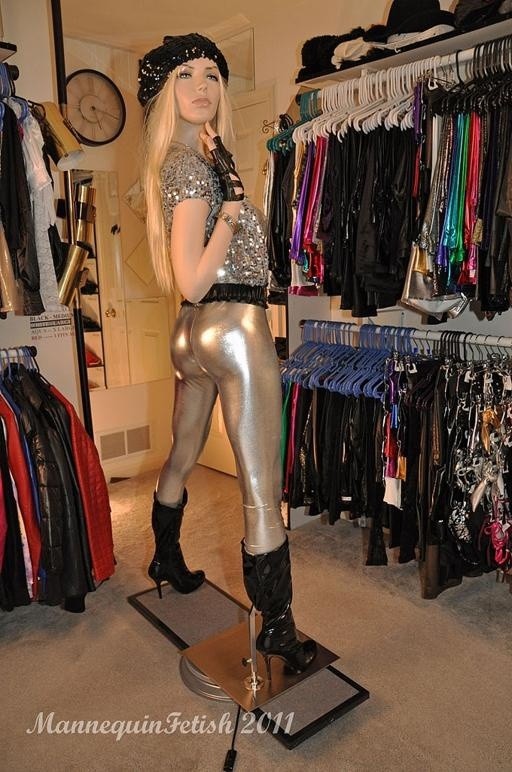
[265,33,511,157]
[1,345,41,384]
[279,319,512,430]
[0,62,47,130]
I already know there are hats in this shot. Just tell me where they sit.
[136,32,230,107]
[362,0,512,50]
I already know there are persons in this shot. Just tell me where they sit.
[137,33,318,680]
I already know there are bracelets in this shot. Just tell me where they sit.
[219,211,240,233]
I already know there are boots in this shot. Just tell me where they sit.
[148,485,206,601]
[239,535,318,682]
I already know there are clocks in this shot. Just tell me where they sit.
[64,69,128,148]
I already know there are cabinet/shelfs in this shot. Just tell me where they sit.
[90,170,177,388]
